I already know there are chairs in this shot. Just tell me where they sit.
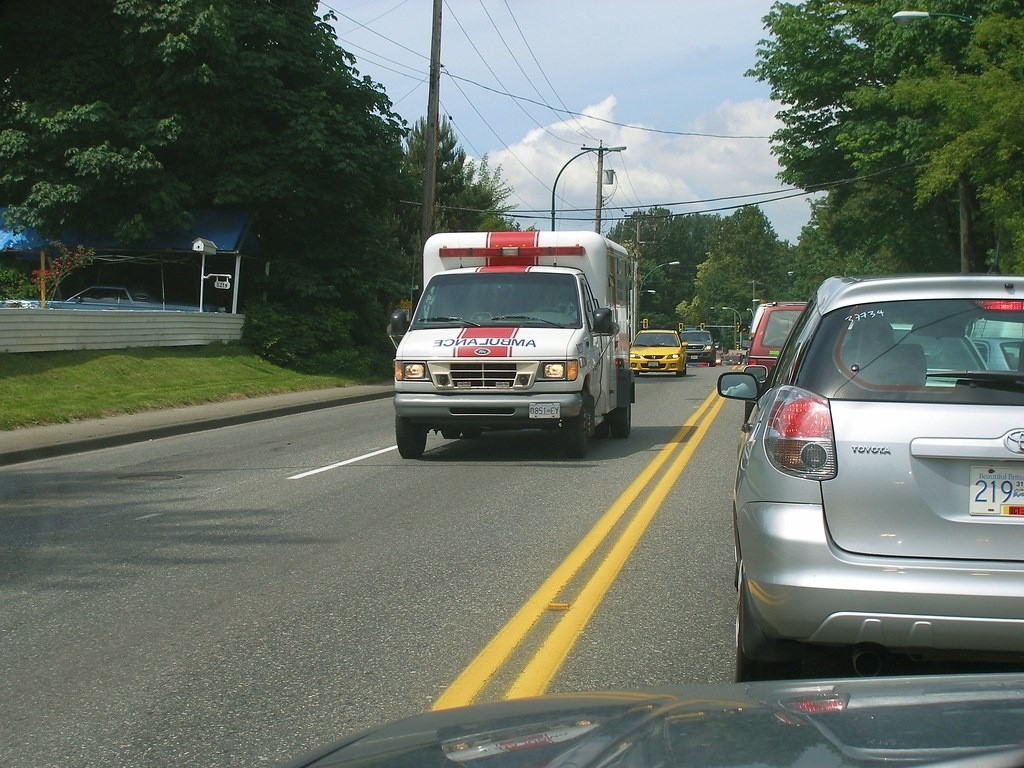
[841,314,928,386]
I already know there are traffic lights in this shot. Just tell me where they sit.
[679,323,683,333]
[736,342,740,350]
[700,323,705,331]
[736,321,740,333]
[643,318,648,329]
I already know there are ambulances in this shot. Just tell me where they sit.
[386,231,638,460]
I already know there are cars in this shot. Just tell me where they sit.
[717,271,1024,681]
[698,351,722,367]
[838,321,990,388]
[271,673,1024,768]
[966,315,1023,373]
[0,285,217,312]
[714,342,719,350]
[630,329,689,377]
[686,363,699,367]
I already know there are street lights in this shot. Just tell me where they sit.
[551,146,627,231]
[892,11,976,28]
[629,289,656,342]
[746,308,755,318]
[638,261,680,329]
[721,306,742,350]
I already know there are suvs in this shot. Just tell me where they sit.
[743,302,806,424]
[679,330,716,367]
